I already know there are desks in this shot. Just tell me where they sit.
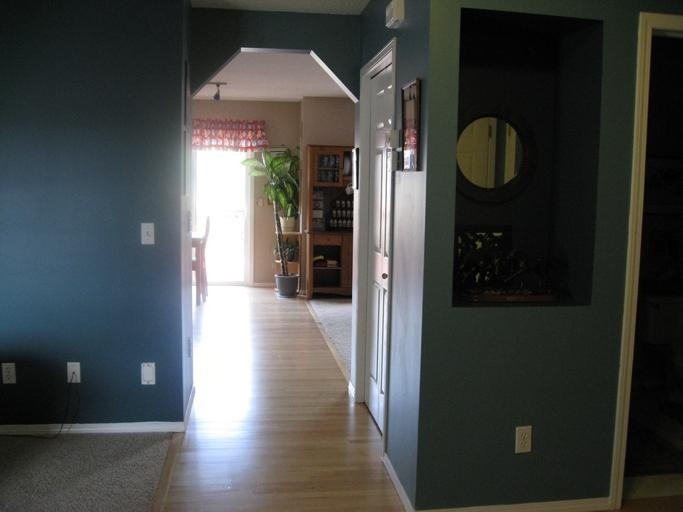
[191,238,202,307]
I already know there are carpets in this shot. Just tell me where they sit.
[303,299,352,383]
[0,432,185,511]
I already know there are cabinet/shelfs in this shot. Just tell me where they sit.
[275,144,353,299]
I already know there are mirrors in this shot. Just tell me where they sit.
[456,102,538,204]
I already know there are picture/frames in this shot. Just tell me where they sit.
[400,78,421,171]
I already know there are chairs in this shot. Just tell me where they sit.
[191,215,210,301]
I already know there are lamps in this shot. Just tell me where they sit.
[208,82,227,100]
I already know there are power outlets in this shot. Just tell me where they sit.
[67,361,80,384]
[1,363,16,384]
[515,426,532,453]
[141,222,155,245]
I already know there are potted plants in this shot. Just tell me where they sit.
[240,146,302,297]
